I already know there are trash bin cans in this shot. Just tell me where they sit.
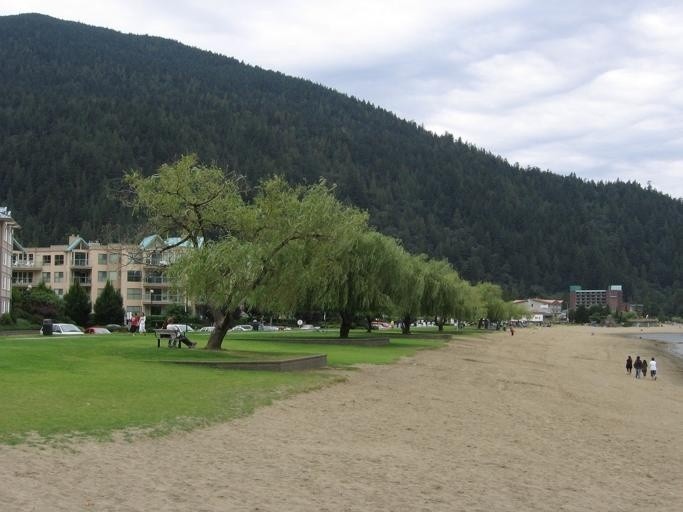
[42,319,53,336]
[251,323,259,331]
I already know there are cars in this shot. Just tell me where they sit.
[231,324,282,331]
[85,327,110,334]
[40,323,83,335]
[167,323,216,332]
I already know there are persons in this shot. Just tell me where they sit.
[626,356,632,374]
[642,360,648,376]
[129,312,147,337]
[634,356,643,379]
[649,356,659,379]
[162,317,198,348]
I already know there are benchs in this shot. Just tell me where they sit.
[153,329,185,349]
